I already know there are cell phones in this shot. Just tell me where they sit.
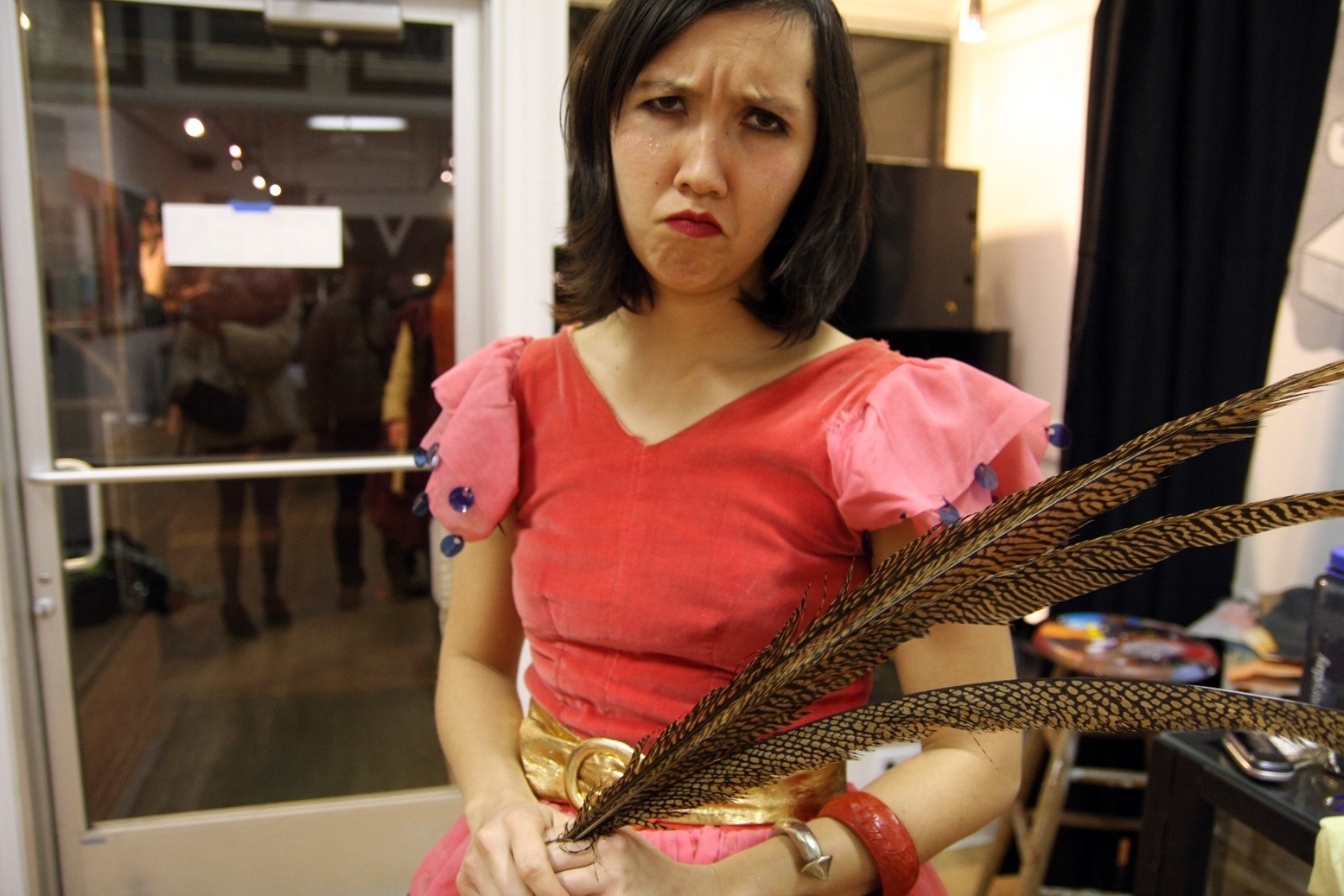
[1223,728,1295,783]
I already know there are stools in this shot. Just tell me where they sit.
[975,612,1219,896]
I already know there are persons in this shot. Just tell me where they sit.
[166,268,293,641]
[408,0,1053,896]
[299,254,440,604]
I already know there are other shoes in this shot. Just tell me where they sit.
[334,571,364,610]
[389,572,431,600]
[220,600,260,643]
[261,593,292,627]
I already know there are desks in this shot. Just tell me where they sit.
[1134,715,1344,896]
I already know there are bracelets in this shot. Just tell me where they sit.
[817,791,920,896]
[770,817,832,881]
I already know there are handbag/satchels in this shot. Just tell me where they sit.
[182,378,249,437]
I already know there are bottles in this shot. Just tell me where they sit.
[1301,546,1344,713]
